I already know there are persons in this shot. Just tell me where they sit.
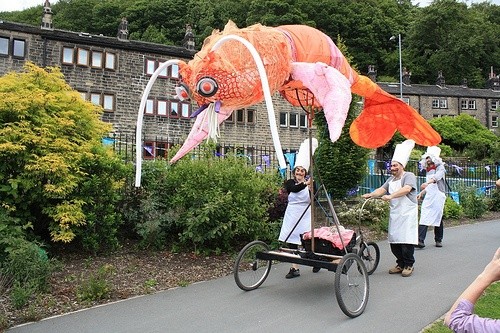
[444,248,500,333]
[361,161,418,276]
[414,156,446,248]
[277,166,321,279]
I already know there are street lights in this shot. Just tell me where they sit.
[389,33,402,101]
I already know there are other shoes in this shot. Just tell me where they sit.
[417,241,425,247]
[313,267,321,273]
[286,267,300,278]
[436,241,443,247]
[389,265,403,274]
[402,265,414,277]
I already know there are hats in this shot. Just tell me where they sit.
[419,145,442,171]
[293,137,318,173]
[391,139,416,168]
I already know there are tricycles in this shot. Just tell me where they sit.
[232,88,380,319]
